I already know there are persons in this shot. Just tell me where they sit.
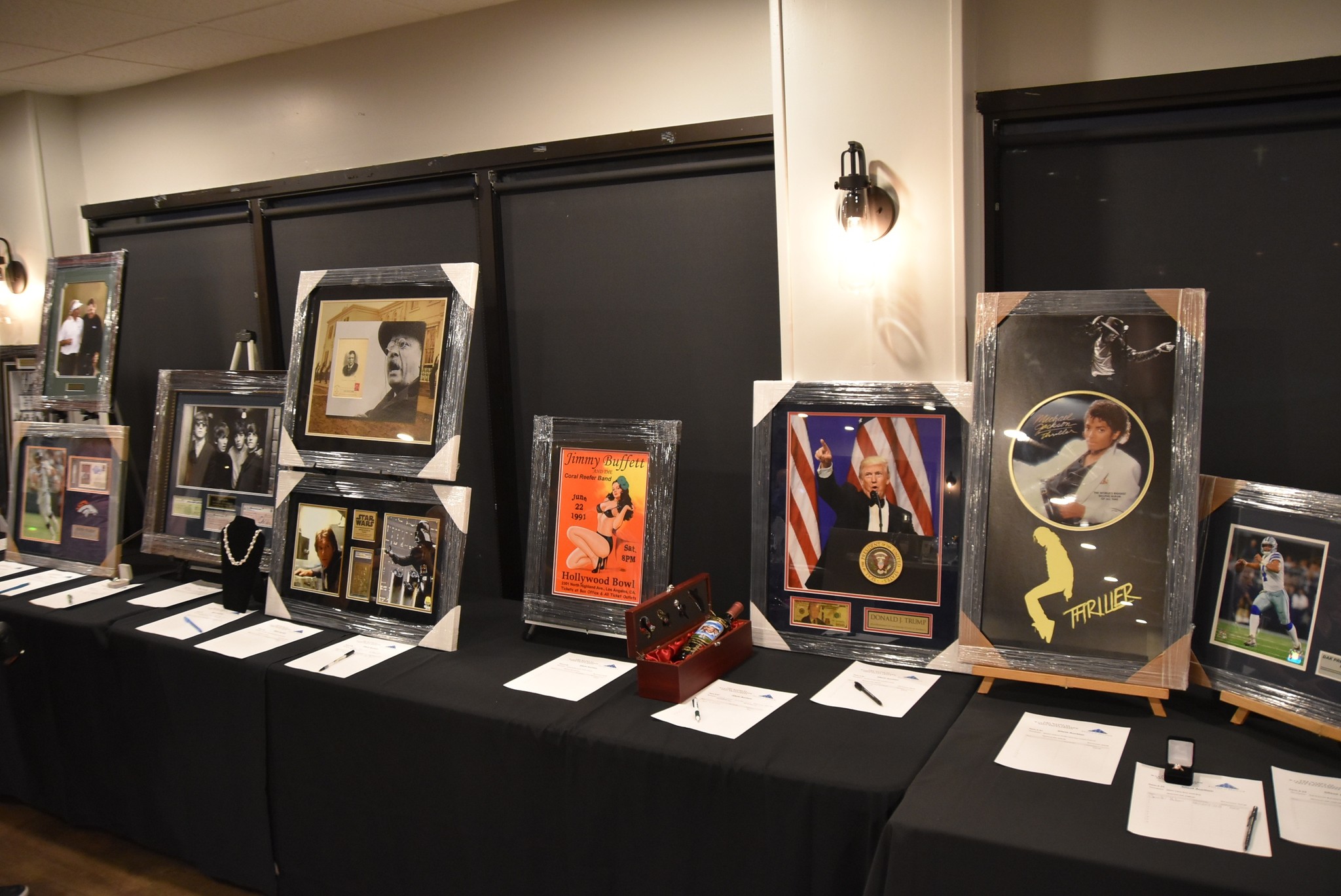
[805,439,918,590]
[294,527,342,593]
[342,350,358,376]
[384,520,437,609]
[27,449,64,542]
[1229,536,1322,661]
[1012,399,1141,528]
[186,411,265,493]
[801,602,826,625]
[58,298,103,376]
[1078,315,1175,397]
[354,321,426,423]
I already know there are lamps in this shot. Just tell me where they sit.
[0,237,26,294]
[833,140,896,242]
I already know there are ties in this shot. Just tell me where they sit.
[320,571,326,591]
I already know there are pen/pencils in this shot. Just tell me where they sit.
[319,650,355,672]
[692,697,701,722]
[184,616,202,633]
[854,681,882,706]
[68,595,72,604]
[0,582,30,594]
[1245,806,1258,850]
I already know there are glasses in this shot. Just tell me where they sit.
[194,421,207,427]
[385,338,406,351]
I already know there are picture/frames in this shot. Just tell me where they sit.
[523,416,683,638]
[749,379,974,670]
[0,345,142,537]
[264,470,472,652]
[277,262,479,481]
[1189,476,1341,727]
[29,250,129,415]
[956,286,1208,693]
[4,421,130,578]
[140,366,287,572]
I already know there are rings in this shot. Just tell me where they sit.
[113,578,120,583]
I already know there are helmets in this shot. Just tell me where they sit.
[1261,536,1277,556]
[35,452,44,462]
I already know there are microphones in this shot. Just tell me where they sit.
[870,490,878,506]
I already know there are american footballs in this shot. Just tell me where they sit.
[1234,560,1244,573]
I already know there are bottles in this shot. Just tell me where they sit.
[672,601,744,662]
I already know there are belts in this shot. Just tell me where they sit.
[1040,488,1053,521]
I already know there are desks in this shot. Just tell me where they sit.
[0,533,1341,896]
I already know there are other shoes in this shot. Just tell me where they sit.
[51,534,56,541]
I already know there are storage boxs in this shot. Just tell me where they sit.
[626,572,754,704]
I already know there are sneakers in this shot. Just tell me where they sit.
[1244,638,1256,647]
[1294,642,1302,656]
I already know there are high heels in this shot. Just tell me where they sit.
[592,557,603,573]
[600,557,608,569]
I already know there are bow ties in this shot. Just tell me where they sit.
[870,499,884,508]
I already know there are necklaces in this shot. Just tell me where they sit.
[223,524,262,566]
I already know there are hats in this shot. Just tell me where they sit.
[378,321,426,356]
[68,300,84,315]
